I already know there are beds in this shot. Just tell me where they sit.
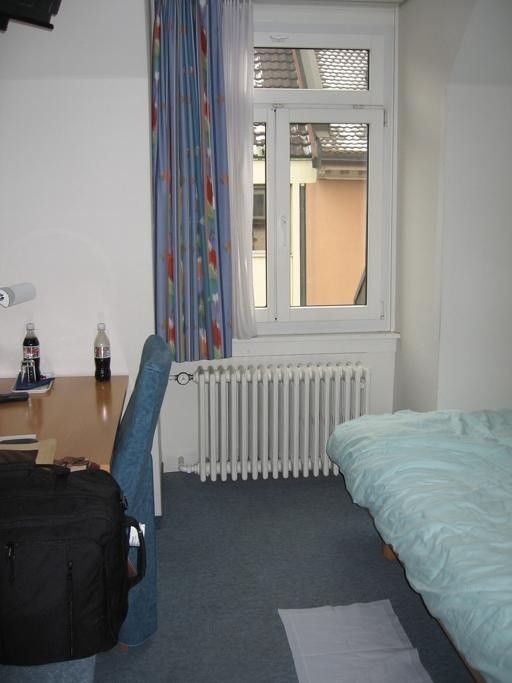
[327,409,512,683]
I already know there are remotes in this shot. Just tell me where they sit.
[0,391,29,403]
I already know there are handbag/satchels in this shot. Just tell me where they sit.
[1,458,146,668]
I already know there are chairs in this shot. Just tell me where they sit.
[111,335,173,650]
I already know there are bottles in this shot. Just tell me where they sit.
[22,322,40,386]
[93,321,112,381]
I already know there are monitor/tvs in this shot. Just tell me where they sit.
[0,0,62,24]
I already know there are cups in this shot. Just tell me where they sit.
[18,359,37,384]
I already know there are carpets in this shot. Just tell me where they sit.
[278,598,434,683]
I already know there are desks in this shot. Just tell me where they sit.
[0,375,128,472]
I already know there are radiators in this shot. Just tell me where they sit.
[169,361,370,482]
